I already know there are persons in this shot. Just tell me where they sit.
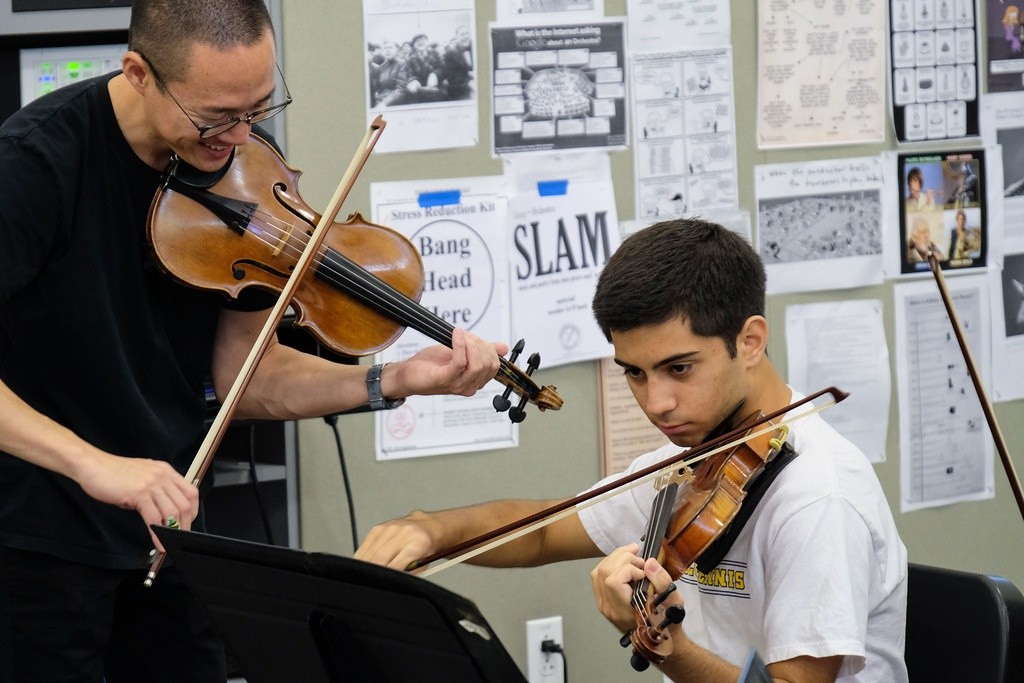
[0,0,510,683]
[905,167,982,262]
[354,219,908,683]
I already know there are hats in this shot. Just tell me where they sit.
[409,33,433,46]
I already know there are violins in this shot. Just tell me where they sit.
[620,411,796,670]
[145,122,566,424]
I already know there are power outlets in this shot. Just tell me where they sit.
[525,615,565,683]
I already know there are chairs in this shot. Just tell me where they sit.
[905,561,1024,683]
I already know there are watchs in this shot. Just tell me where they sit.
[365,362,405,411]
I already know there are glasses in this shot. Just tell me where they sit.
[131,47,292,140]
